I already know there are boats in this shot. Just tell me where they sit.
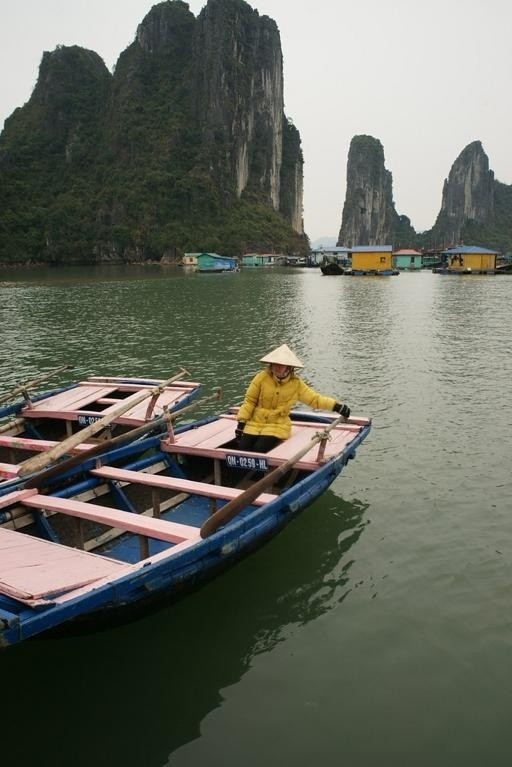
[0,406,370,649]
[0,376,201,491]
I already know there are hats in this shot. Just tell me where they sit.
[258,343,304,368]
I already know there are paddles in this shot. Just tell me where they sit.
[18,366,192,475]
[201,417,347,539]
[25,386,220,490]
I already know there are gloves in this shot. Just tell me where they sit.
[333,404,350,417]
[235,422,245,439]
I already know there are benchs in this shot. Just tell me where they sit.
[0,435,279,562]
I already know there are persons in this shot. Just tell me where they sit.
[233,342,350,451]
